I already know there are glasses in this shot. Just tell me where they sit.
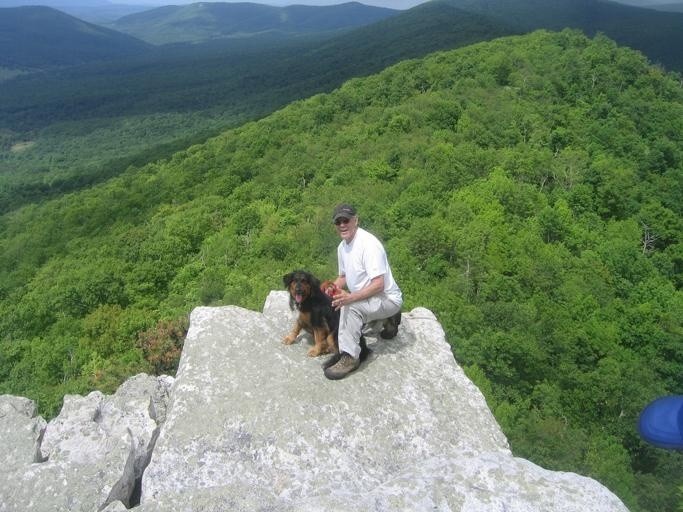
[335,220,349,225]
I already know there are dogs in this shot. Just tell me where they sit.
[282,270,369,371]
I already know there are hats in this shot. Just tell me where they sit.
[333,204,355,224]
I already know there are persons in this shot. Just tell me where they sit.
[323,204,403,380]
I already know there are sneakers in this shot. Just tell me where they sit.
[325,353,361,379]
[381,311,401,339]
